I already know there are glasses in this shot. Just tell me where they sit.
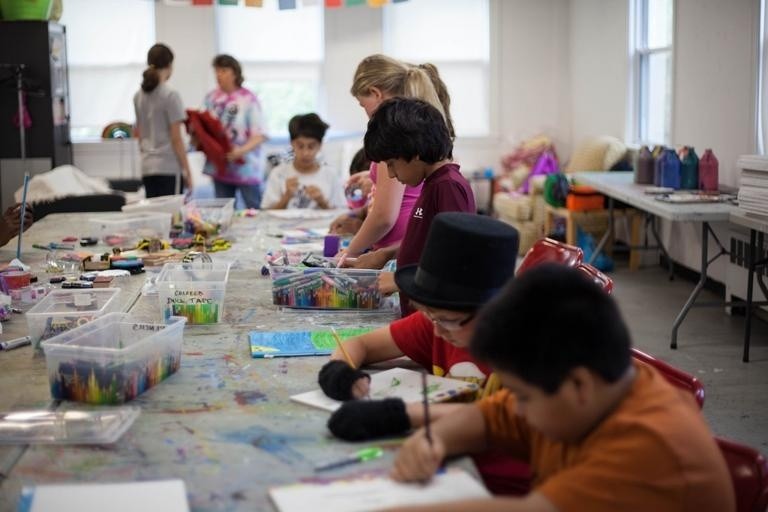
[424,311,473,331]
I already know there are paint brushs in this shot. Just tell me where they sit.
[329,326,372,402]
[421,369,432,444]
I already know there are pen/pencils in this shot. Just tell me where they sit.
[262,248,383,310]
[50,275,96,288]
[314,447,384,472]
[0,335,32,351]
[50,338,182,406]
[32,237,78,250]
[36,317,87,350]
[265,233,284,238]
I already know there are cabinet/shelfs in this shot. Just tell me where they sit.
[0,18,72,170]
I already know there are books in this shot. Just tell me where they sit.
[247,326,376,359]
[266,464,497,512]
[288,365,482,412]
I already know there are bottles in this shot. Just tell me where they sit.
[632,143,719,191]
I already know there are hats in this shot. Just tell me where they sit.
[393,211,519,313]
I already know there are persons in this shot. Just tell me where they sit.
[258,112,348,211]
[327,52,476,318]
[132,42,193,196]
[317,211,536,497]
[189,55,266,211]
[369,261,736,512]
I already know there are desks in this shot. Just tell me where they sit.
[543,201,643,273]
[573,170,767,364]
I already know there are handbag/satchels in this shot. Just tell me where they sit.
[566,184,604,212]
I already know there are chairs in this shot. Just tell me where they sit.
[492,134,627,258]
